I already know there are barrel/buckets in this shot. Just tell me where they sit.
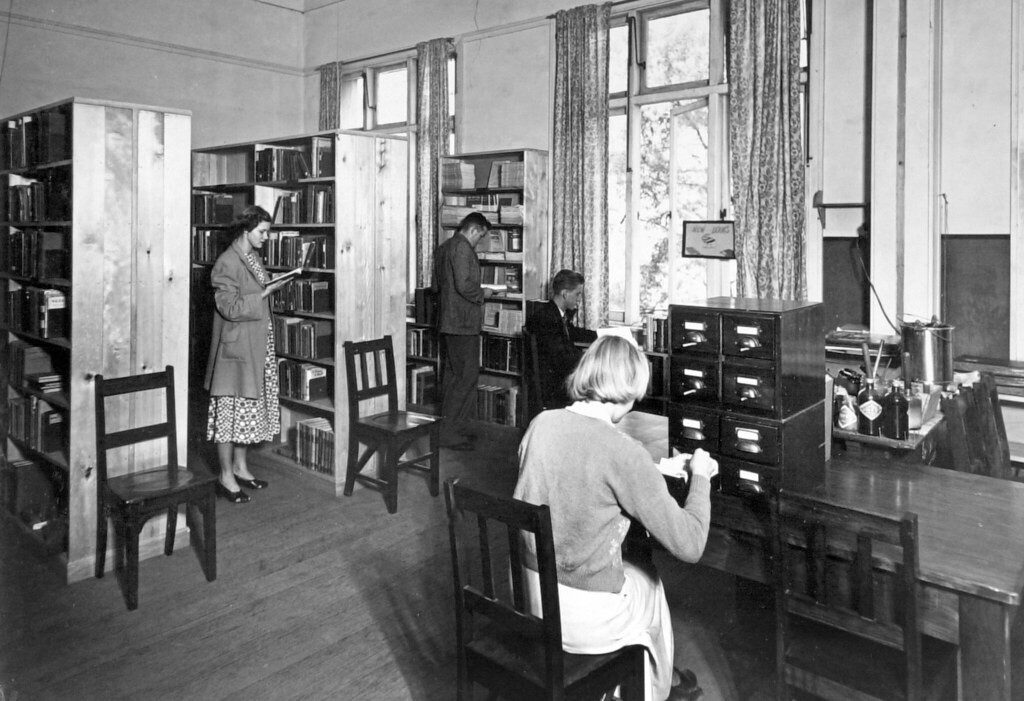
[896,321,955,384]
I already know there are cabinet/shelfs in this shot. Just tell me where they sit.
[189,129,409,494]
[667,296,827,504]
[405,149,551,431]
[574,341,668,414]
[0,96,192,584]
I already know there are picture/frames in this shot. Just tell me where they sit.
[681,220,735,260]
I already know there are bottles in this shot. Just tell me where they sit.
[857,379,882,435]
[508,229,521,252]
[883,384,909,441]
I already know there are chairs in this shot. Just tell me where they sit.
[342,335,442,513]
[443,475,645,701]
[94,365,218,610]
[941,374,1024,485]
[766,482,959,701]
[519,323,566,431]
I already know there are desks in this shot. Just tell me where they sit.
[831,413,946,470]
[614,410,1024,701]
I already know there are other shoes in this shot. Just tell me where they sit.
[468,435,477,440]
[448,442,476,450]
[670,666,703,700]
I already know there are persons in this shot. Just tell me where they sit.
[203,205,284,503]
[526,269,598,406]
[509,333,719,701]
[431,212,496,451]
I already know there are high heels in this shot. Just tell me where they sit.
[234,474,269,490]
[214,477,251,503]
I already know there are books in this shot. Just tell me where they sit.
[596,328,638,348]
[265,267,303,285]
[480,284,507,292]
[643,314,667,399]
[953,355,1024,398]
[0,109,71,554]
[648,453,718,489]
[824,333,902,358]
[188,134,335,475]
[407,161,524,428]
